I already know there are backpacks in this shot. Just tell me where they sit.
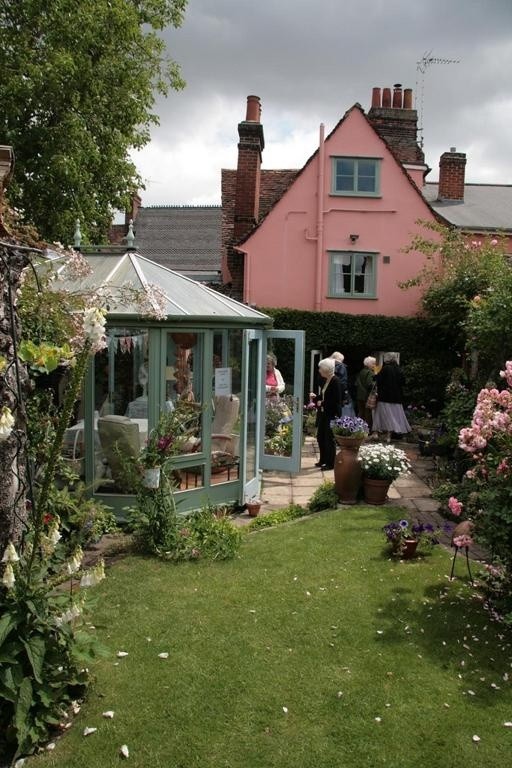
[365,390,378,410]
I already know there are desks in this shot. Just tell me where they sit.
[66,416,148,449]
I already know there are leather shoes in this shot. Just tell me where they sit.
[315,461,333,471]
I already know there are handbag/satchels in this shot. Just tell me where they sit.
[275,402,293,424]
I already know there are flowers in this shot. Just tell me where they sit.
[405,404,442,426]
[449,359,512,574]
[0,241,167,366]
[381,518,454,555]
[330,415,369,439]
[356,442,411,478]
[395,213,512,358]
[303,402,318,426]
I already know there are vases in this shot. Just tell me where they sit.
[413,425,439,440]
[304,416,317,433]
[333,434,364,504]
[401,539,419,558]
[364,478,392,504]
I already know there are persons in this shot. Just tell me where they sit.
[307,356,341,471]
[371,350,414,444]
[331,350,348,408]
[265,351,286,400]
[353,356,378,427]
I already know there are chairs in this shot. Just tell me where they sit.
[96,392,241,490]
